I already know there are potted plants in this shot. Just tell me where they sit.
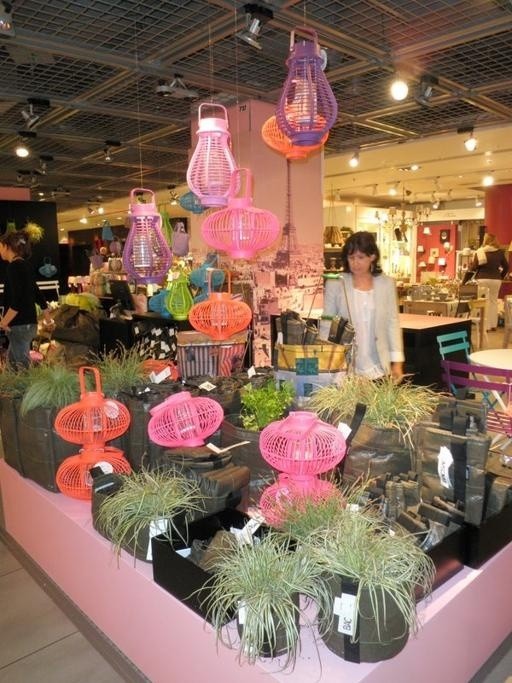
[0,342,148,492]
[87,461,211,566]
[307,373,424,486]
[150,375,248,416]
[222,378,296,468]
[182,469,424,661]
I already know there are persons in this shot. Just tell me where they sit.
[459,232,509,333]
[0,230,56,370]
[316,232,405,385]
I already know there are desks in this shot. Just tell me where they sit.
[402,297,489,352]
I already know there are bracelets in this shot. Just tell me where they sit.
[43,319,55,326]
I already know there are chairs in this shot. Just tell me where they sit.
[435,328,512,469]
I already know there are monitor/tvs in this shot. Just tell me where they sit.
[108,280,136,311]
